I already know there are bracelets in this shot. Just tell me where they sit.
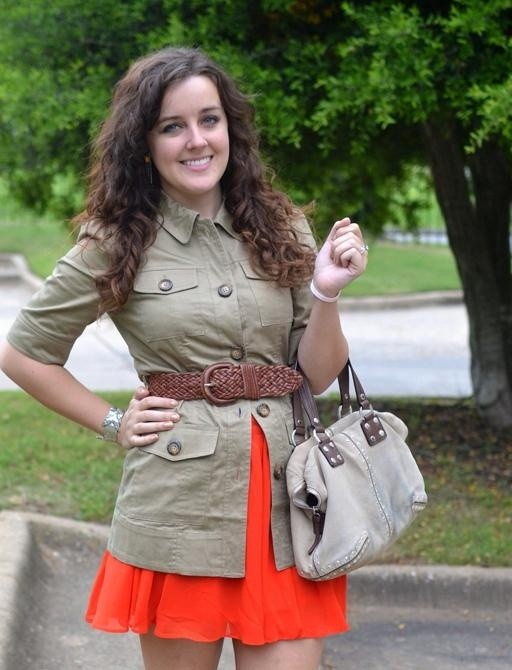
[308,280,342,304]
[98,406,130,443]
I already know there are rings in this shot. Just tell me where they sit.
[361,244,369,255]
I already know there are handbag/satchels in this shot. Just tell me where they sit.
[284,350,429,582]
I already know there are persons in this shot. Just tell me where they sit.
[0,40,373,669]
